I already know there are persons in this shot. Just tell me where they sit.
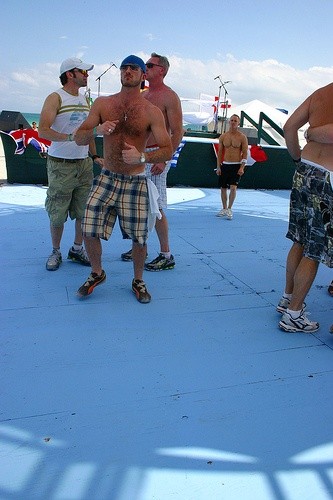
[277,81,333,313]
[38,57,104,271]
[74,52,183,303]
[32,122,38,131]
[215,113,248,216]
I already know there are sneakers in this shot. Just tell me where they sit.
[46,251,62,270]
[278,309,319,333]
[145,253,175,272]
[276,296,306,315]
[76,270,106,296]
[121,249,148,261]
[66,247,91,266]
[132,278,151,303]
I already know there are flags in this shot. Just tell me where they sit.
[0,129,267,167]
[212,97,231,113]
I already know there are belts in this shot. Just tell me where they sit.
[48,156,84,163]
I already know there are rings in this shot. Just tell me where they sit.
[107,129,112,132]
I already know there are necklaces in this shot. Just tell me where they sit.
[121,111,132,121]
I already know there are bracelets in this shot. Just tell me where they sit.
[67,135,73,141]
[242,159,247,163]
[92,153,100,159]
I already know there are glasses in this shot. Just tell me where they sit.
[120,65,144,72]
[72,68,87,75]
[145,63,164,68]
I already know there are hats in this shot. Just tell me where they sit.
[60,58,94,75]
[120,55,146,89]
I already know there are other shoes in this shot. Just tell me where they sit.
[226,209,233,220]
[216,208,227,217]
[328,282,333,296]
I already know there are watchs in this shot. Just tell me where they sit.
[139,152,147,164]
[304,132,310,140]
